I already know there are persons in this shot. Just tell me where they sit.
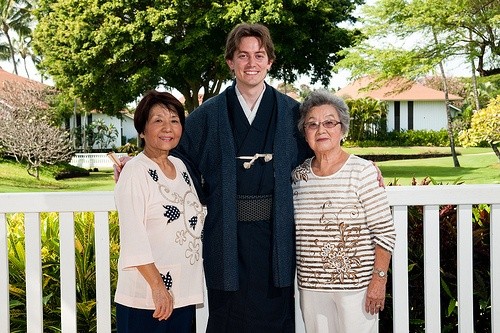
[286,88,397,333]
[114,21,386,333]
[114,90,206,333]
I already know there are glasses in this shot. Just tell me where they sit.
[304,119,343,129]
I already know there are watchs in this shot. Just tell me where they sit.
[372,269,387,277]
